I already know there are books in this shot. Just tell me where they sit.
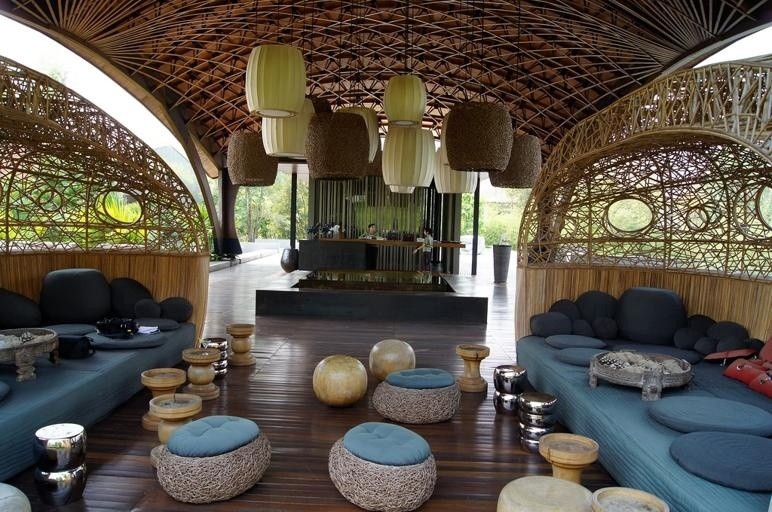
[137,326,161,335]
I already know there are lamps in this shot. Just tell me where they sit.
[228,132,278,187]
[381,127,436,187]
[261,98,317,156]
[244,43,307,119]
[334,107,380,163]
[440,100,513,173]
[434,146,478,194]
[304,112,370,181]
[488,134,541,188]
[383,75,427,125]
[288,97,332,159]
[389,184,416,194]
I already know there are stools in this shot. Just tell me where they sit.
[329,422,437,512]
[201,338,228,380]
[493,365,527,416]
[0,482,31,512]
[158,415,271,504]
[517,391,557,453]
[34,423,88,507]
[497,476,594,512]
[372,368,460,425]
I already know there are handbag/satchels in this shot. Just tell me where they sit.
[724,333,771,399]
[95,317,139,339]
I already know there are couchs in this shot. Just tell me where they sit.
[0,250,210,482]
[515,266,772,512]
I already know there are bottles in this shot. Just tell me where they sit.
[641,370,663,402]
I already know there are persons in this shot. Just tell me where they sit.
[358,224,387,269]
[359,272,383,282]
[413,227,434,271]
[416,271,433,284]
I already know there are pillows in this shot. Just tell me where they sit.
[529,286,764,359]
[0,268,193,321]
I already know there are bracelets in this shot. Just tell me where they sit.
[416,248,419,252]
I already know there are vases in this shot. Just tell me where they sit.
[281,249,298,273]
[493,244,512,282]
